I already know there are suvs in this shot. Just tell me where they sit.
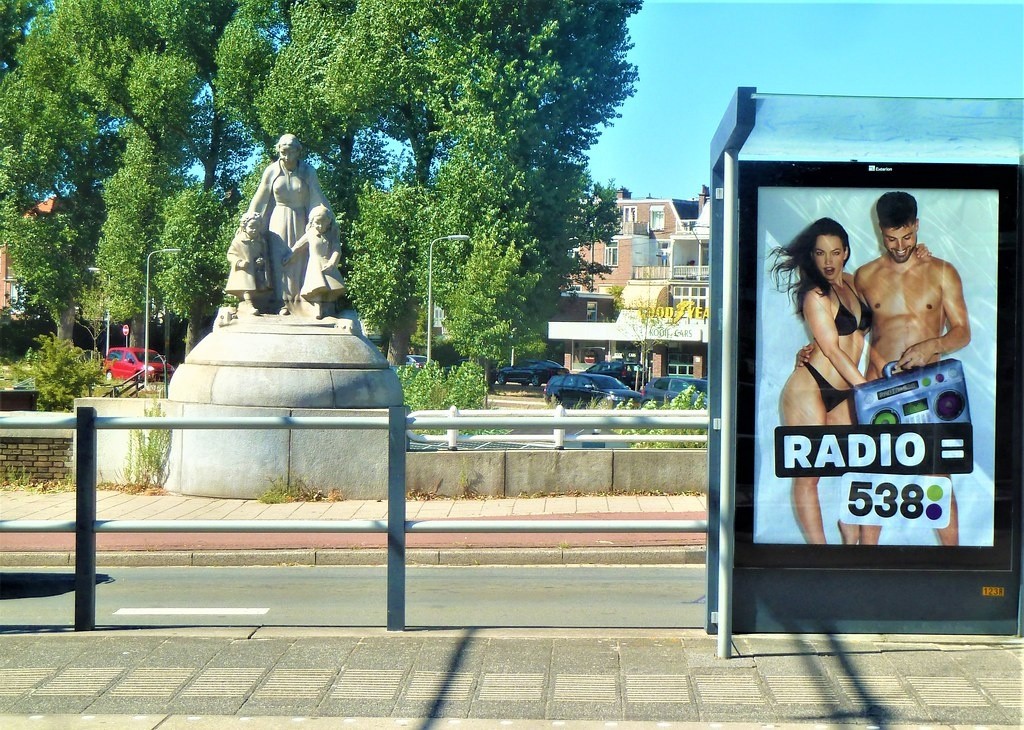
[542,373,641,409]
[584,360,649,391]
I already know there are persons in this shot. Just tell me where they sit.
[793,189,971,546]
[224,133,348,320]
[769,217,936,546]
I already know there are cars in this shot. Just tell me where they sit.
[405,354,435,370]
[497,358,571,391]
[639,377,708,409]
[104,347,175,383]
[443,358,497,386]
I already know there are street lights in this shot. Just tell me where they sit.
[88,268,111,359]
[141,248,182,391]
[426,236,472,371]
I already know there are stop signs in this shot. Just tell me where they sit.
[123,325,130,336]
